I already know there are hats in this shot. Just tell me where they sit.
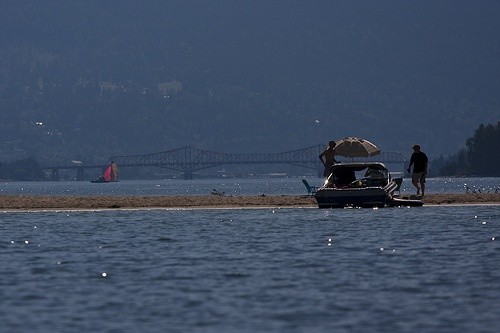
[412,144,421,149]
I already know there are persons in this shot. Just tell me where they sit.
[407,144,429,195]
[319,140,341,181]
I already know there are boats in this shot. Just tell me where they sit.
[315,161,423,206]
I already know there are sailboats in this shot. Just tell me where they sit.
[90,161,120,183]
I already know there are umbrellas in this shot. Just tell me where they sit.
[325,135,381,163]
